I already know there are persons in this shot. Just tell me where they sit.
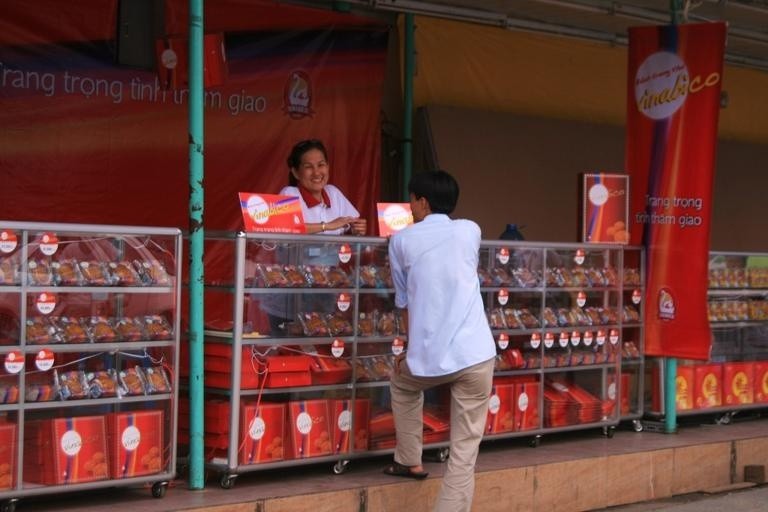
[257,138,368,338]
[382,169,497,512]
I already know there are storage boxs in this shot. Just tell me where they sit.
[576,173,629,244]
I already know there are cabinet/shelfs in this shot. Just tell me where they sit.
[0,219,185,512]
[176,227,623,490]
[644,248,768,422]
[602,244,644,437]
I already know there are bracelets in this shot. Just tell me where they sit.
[321,221,326,232]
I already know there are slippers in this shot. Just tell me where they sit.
[381,461,430,479]
